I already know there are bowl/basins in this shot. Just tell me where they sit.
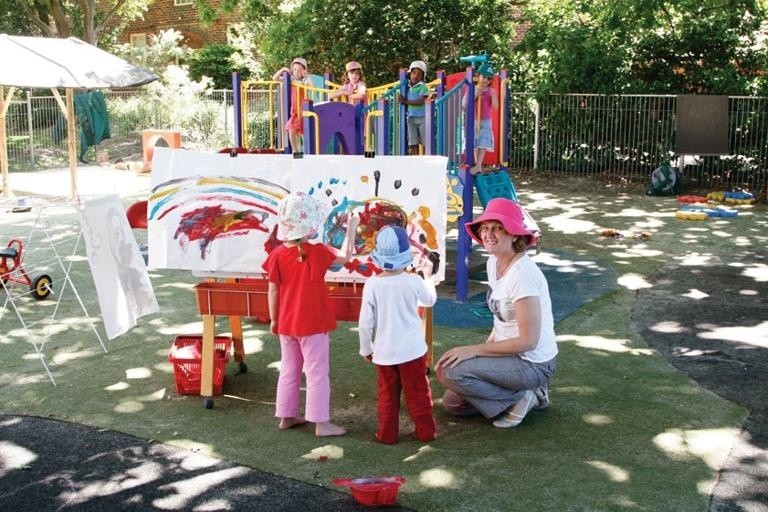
[348,475,407,507]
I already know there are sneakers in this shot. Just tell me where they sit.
[493,383,548,428]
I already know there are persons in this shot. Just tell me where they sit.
[271,57,313,155]
[435,196,559,430]
[260,192,361,438]
[328,61,370,154]
[461,64,499,176]
[377,61,429,154]
[357,224,438,445]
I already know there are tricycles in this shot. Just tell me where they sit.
[0,236,54,300]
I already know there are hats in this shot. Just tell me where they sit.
[464,198,535,252]
[477,64,494,79]
[277,192,329,242]
[346,61,361,72]
[291,58,307,73]
[369,226,413,271]
[331,477,406,506]
[407,60,427,79]
